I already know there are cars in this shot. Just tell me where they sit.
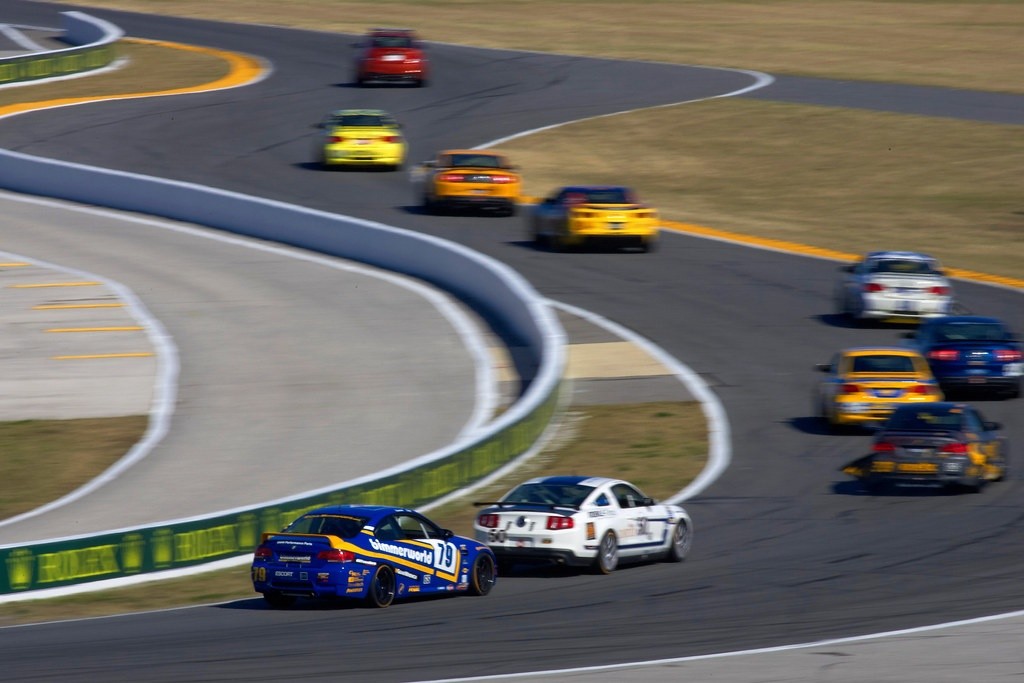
[811,347,940,431]
[869,400,1008,489]
[901,313,1024,398]
[530,187,659,251]
[315,110,413,169]
[416,148,523,212]
[473,475,695,574]
[251,504,498,606]
[349,28,429,88]
[828,252,957,323]
